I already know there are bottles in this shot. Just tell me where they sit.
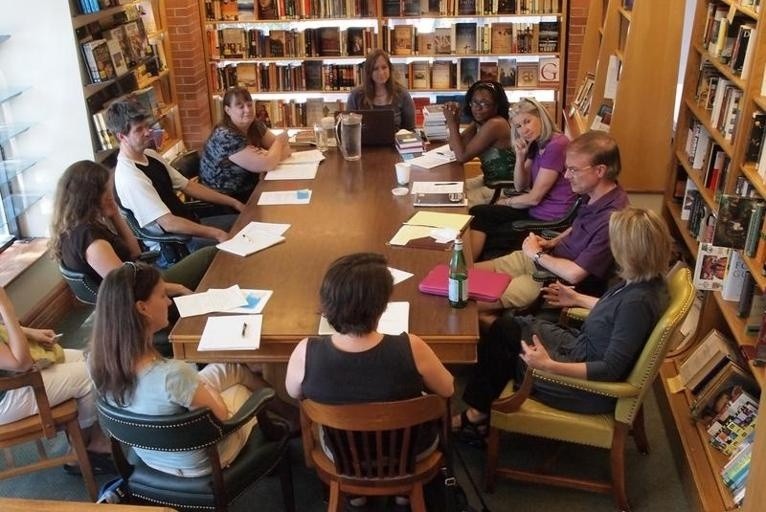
[447,237,468,309]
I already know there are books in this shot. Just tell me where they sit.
[384,209,476,252]
[413,192,467,206]
[68,0,174,151]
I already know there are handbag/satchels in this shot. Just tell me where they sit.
[423,467,468,512]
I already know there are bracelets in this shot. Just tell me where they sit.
[534,251,543,264]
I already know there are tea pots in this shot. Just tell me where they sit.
[333,112,363,162]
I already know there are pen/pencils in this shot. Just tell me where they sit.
[551,285,578,289]
[242,322,246,335]
[51,334,63,340]
[437,152,452,157]
[242,234,252,245]
[434,183,458,185]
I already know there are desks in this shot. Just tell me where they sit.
[168,140,481,457]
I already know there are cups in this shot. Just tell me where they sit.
[395,162,411,184]
[321,117,336,147]
[314,125,329,153]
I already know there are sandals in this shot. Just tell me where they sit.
[450,409,489,438]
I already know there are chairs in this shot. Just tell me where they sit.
[0,129,293,510]
[295,389,465,511]
[336,99,631,335]
[480,255,696,509]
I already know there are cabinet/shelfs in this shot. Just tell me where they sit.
[381,0,569,132]
[659,1,766,512]
[69,0,182,172]
[0,31,46,234]
[197,1,379,140]
[563,1,687,193]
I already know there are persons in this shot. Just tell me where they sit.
[90,261,302,479]
[472,130,631,323]
[0,283,114,478]
[54,161,220,299]
[105,99,245,269]
[470,95,579,263]
[441,79,530,209]
[284,251,455,512]
[449,207,677,442]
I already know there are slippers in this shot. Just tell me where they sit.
[63,449,117,475]
